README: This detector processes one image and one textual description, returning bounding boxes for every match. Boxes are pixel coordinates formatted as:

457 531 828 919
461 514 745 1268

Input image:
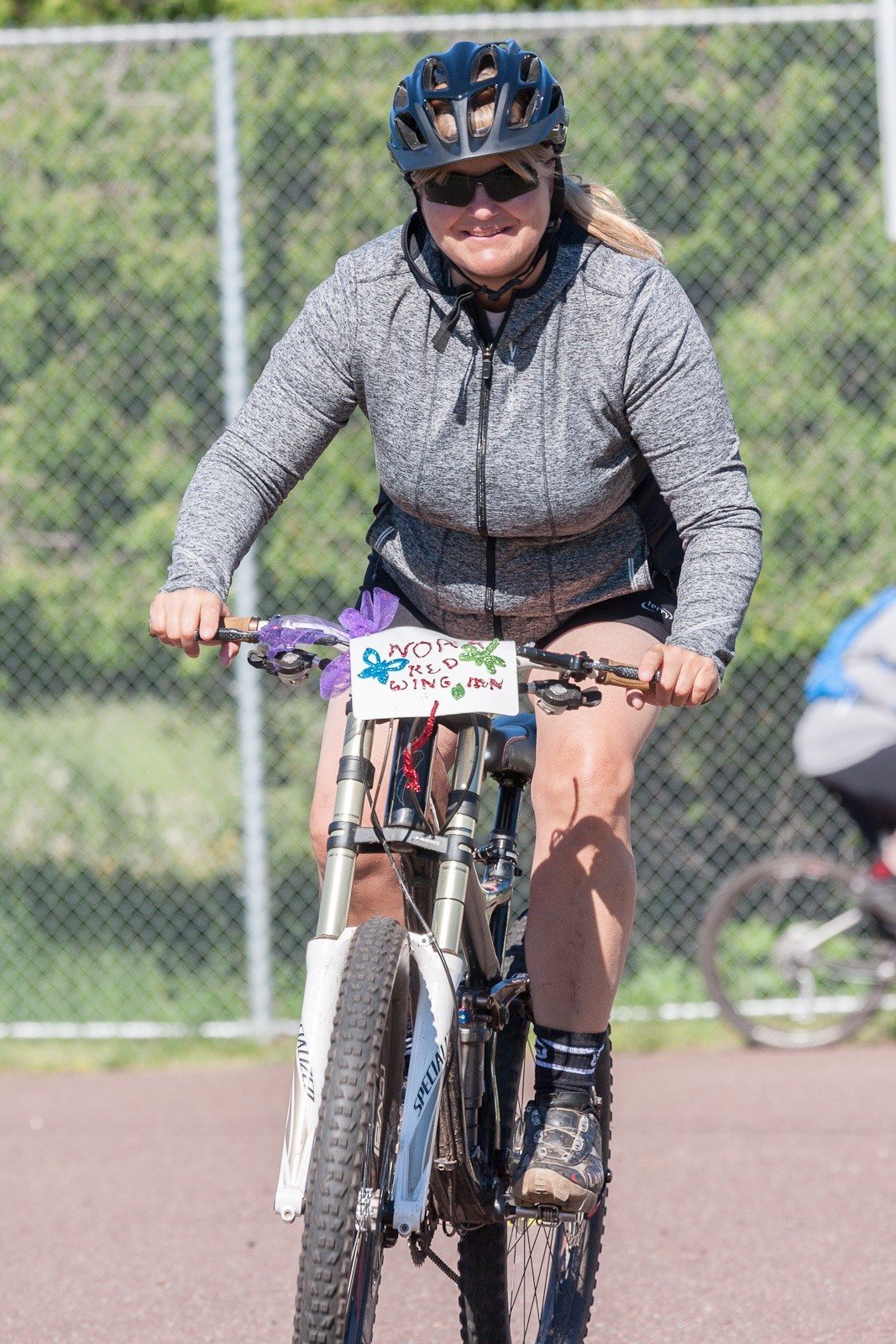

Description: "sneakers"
858 869 896 939
509 1091 606 1214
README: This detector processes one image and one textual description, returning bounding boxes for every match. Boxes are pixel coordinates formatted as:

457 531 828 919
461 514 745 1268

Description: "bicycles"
196 613 663 1344
699 852 896 1056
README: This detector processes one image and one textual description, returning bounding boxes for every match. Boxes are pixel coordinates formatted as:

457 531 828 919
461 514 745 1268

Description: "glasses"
424 156 556 207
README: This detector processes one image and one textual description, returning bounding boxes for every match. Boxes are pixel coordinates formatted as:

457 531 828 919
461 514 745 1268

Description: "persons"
149 41 763 1219
794 588 896 924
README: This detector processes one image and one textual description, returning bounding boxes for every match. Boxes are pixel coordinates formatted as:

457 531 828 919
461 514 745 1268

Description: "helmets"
384 39 570 176
